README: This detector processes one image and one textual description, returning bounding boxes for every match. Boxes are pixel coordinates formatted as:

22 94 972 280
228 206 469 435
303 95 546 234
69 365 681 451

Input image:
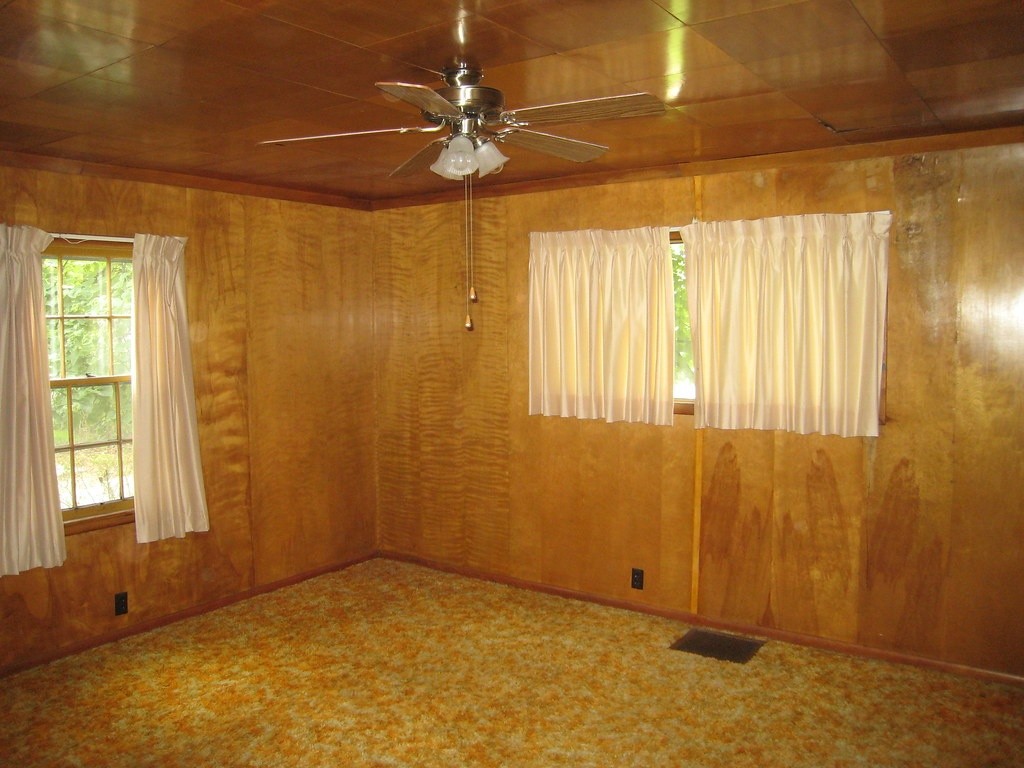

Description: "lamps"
427 119 509 184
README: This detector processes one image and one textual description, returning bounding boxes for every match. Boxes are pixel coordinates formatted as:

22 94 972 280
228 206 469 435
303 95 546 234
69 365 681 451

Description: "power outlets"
632 568 644 590
114 592 128 616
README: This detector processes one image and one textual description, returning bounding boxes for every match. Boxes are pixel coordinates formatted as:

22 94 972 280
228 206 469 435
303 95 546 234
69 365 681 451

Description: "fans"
245 69 670 182
